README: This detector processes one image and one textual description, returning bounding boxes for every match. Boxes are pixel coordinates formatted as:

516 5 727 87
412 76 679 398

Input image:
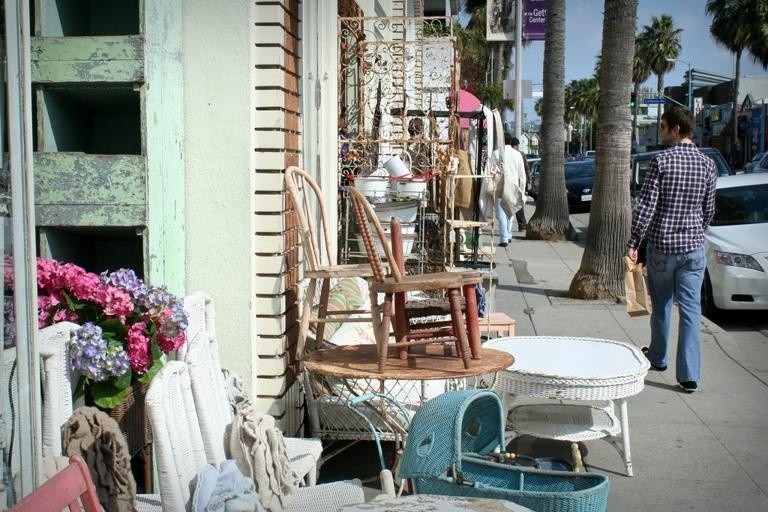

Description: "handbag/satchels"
623 256 649 317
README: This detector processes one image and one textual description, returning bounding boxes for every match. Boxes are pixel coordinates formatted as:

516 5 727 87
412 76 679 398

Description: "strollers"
349 391 612 512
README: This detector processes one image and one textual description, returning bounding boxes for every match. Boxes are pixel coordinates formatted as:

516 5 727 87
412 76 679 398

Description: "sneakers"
678 382 697 392
519 224 527 232
641 347 666 371
499 239 511 247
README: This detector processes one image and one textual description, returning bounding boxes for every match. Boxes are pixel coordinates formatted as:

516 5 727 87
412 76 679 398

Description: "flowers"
3 246 190 456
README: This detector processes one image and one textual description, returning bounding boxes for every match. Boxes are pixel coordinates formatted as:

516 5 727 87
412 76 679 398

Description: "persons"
405 118 430 168
483 133 530 247
627 104 718 391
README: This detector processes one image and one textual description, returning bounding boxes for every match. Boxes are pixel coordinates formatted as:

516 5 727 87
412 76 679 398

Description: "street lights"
569 106 593 150
666 58 692 113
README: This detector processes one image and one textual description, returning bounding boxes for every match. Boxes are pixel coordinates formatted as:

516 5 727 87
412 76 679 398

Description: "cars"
632 147 734 196
524 153 541 201
701 172 768 314
563 160 595 211
563 150 595 159
743 153 766 172
755 151 768 172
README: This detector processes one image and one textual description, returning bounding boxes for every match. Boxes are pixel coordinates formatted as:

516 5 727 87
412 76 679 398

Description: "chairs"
281 143 652 478
182 326 316 490
141 359 219 510
176 289 326 468
